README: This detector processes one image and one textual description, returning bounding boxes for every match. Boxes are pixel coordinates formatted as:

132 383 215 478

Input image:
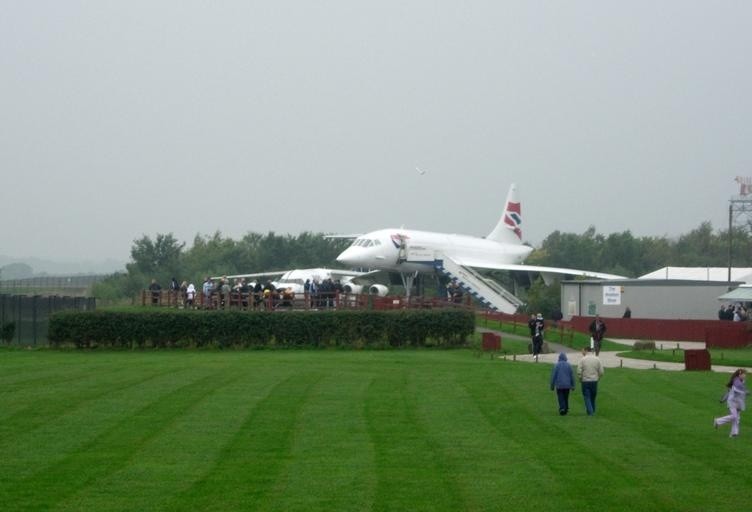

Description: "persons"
711 367 751 438
551 305 563 321
443 279 464 303
147 278 164 306
586 314 608 357
528 312 545 359
530 313 545 337
717 302 751 322
167 275 345 310
574 346 606 417
550 351 577 415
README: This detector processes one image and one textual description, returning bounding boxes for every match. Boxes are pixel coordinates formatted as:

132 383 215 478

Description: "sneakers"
729 434 739 437
713 418 719 430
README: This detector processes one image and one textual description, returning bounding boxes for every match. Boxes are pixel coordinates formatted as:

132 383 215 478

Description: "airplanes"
207 268 395 307
321 179 637 315
